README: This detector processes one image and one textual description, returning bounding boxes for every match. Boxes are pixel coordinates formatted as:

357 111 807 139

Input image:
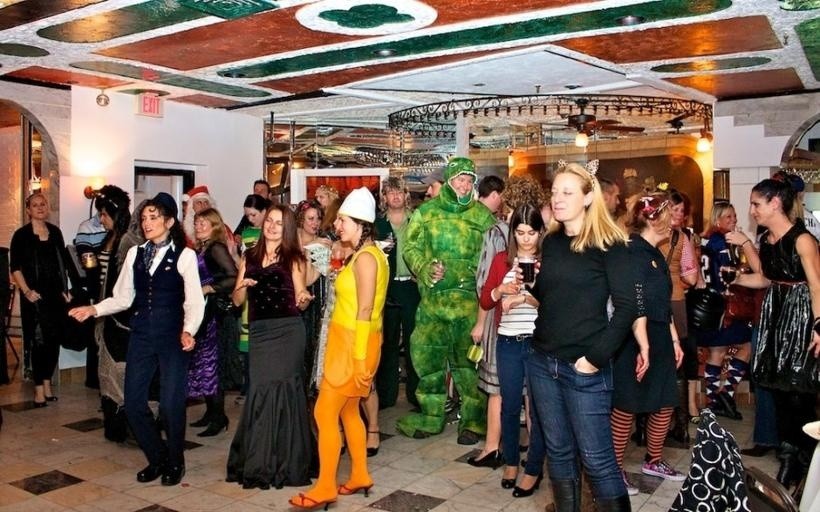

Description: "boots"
666 380 690 444
630 412 647 446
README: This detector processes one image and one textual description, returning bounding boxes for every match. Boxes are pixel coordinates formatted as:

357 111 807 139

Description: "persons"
1 150 820 510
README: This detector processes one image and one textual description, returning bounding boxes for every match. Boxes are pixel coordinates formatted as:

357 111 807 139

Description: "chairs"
741 467 799 512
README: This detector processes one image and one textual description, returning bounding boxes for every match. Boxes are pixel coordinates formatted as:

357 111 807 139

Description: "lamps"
84 175 105 199
567 122 597 147
696 117 713 152
508 150 514 168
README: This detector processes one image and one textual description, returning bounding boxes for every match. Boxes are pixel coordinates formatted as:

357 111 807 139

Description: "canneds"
83 253 93 268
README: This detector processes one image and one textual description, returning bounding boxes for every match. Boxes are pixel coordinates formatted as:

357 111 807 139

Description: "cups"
385 231 393 251
433 259 444 280
82 252 98 268
731 226 743 233
519 255 536 284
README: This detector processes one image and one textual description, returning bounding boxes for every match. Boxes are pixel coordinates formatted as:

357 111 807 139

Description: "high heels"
338 483 374 497
189 409 229 437
467 448 542 498
288 494 337 511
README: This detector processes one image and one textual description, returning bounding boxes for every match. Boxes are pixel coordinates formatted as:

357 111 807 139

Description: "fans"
530 97 644 136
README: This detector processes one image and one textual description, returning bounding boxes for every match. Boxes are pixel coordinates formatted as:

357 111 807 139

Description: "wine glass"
245 239 255 249
739 250 751 272
330 247 344 285
721 265 734 298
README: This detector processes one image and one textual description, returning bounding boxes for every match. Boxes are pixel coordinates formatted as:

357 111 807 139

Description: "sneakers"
621 470 638 496
457 430 479 444
642 459 686 481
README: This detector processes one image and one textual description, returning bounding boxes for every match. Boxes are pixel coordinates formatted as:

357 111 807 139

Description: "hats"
338 186 376 224
153 192 178 215
420 167 445 184
184 186 209 201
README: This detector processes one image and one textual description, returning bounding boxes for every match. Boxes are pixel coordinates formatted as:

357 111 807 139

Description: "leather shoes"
740 440 809 489
366 430 381 457
689 391 742 424
45 395 57 401
162 464 185 486
136 465 167 482
33 401 46 407
